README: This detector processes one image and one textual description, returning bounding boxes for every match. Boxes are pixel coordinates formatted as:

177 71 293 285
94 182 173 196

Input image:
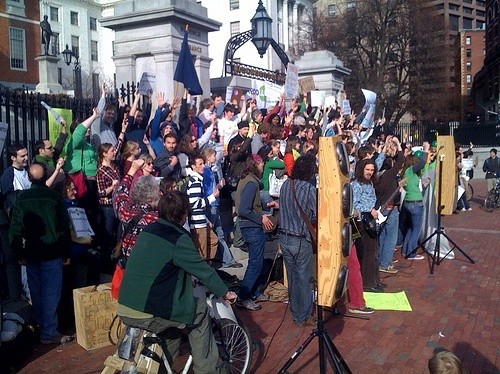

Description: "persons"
116 191 237 374
187 155 220 266
152 134 182 185
0 89 475 342
278 155 317 326
227 121 252 159
482 149 500 206
62 107 101 237
233 153 278 309
428 347 463 374
375 137 406 273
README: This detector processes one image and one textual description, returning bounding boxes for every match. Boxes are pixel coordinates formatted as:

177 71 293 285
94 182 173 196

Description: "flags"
173 30 203 95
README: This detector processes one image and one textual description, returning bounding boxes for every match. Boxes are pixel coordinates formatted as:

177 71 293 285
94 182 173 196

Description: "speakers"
436 136 458 215
318 135 350 308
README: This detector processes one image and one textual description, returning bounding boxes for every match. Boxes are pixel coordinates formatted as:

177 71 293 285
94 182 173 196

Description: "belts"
405 200 422 203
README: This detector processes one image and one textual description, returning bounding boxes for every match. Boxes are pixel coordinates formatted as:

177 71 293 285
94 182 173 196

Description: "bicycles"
484 174 500 210
118 294 254 374
465 182 475 202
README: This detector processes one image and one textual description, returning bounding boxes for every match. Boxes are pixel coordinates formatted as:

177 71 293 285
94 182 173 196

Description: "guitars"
361 177 407 238
308 221 318 255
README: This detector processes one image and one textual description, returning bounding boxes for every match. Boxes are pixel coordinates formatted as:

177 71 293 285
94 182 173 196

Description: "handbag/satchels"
66 168 87 197
268 169 288 197
263 281 289 302
224 163 238 188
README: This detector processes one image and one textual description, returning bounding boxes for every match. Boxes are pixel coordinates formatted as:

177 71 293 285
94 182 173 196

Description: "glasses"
191 138 197 143
147 162 154 166
46 146 52 150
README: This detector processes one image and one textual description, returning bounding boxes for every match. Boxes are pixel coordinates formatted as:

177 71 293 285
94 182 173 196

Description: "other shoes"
377 282 387 288
39 333 73 346
348 306 375 314
228 261 243 268
251 293 268 303
364 287 384 293
392 256 398 263
235 296 262 310
460 207 472 211
379 264 398 274
408 253 425 260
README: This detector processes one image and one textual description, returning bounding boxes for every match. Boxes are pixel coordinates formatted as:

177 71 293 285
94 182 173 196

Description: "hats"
237 121 249 129
432 140 437 146
328 110 340 122
490 148 497 155
414 149 428 162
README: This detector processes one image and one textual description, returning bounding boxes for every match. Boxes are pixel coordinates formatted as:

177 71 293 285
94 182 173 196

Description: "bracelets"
120 131 126 134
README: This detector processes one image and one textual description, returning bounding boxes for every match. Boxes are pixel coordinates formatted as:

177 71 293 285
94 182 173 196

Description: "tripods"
404 156 475 274
277 305 352 374
